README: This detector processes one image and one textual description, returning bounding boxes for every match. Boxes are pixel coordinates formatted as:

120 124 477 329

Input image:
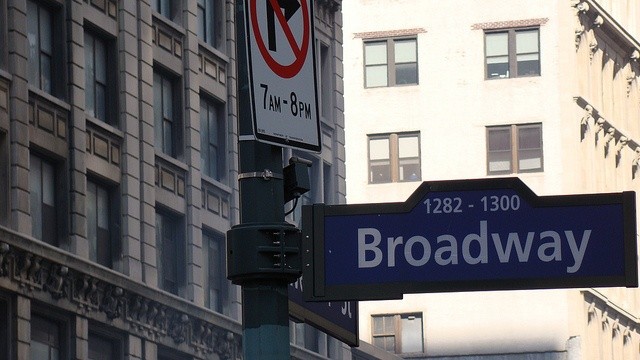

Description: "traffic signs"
286 276 359 348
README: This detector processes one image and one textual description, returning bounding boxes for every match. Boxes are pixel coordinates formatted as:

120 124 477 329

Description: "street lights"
302 177 638 302
